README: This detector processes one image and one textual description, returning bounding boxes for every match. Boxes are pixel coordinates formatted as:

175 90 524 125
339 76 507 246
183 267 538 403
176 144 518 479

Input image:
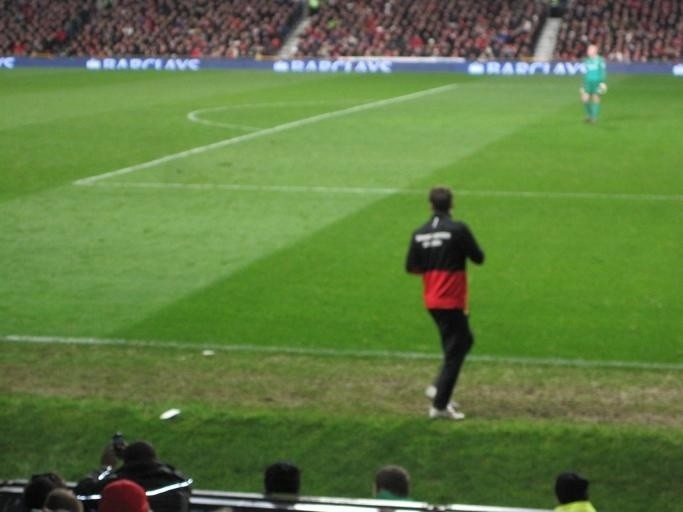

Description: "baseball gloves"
597 82 607 98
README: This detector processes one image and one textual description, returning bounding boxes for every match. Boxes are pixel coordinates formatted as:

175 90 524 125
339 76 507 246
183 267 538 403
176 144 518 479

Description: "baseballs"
203 349 214 357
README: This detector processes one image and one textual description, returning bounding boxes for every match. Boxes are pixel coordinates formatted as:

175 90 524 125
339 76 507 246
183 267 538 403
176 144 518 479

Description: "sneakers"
424 385 466 421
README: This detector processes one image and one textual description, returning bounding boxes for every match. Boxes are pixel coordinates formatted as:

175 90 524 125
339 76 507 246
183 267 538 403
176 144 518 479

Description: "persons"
404 183 485 420
552 467 598 510
262 460 301 494
0 438 193 511
576 44 607 125
0 0 683 65
370 463 412 499
